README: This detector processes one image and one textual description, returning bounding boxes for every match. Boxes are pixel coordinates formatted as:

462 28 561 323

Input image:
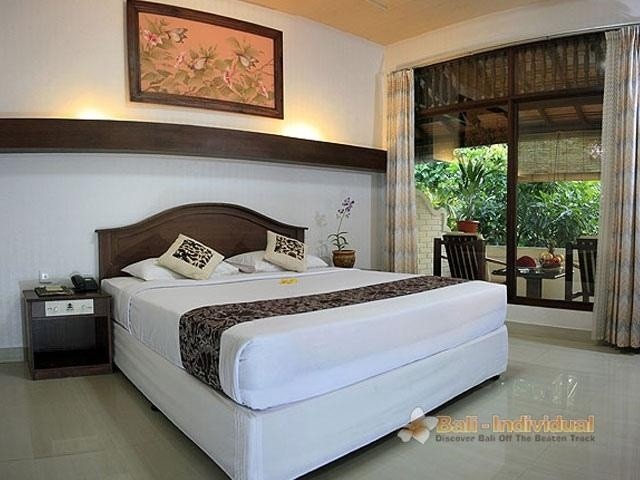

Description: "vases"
332 251 356 268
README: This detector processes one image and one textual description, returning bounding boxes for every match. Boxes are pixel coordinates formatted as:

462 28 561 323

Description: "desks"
490 264 575 299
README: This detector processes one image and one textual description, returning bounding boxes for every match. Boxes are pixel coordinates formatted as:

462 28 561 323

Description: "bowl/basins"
540 263 562 273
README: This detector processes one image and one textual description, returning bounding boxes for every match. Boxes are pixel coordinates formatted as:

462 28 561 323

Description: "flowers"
326 196 356 250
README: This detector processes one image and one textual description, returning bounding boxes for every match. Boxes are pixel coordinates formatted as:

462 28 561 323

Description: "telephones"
71 275 98 290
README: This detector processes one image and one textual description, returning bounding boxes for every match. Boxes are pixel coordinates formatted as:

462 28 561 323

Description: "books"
35 284 74 297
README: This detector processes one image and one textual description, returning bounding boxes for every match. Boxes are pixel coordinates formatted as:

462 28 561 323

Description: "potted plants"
452 156 497 232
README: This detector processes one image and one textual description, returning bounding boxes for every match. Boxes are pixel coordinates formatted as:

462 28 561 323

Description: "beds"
92 202 510 480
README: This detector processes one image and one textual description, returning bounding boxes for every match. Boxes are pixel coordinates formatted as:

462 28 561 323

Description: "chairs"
432 232 508 285
564 238 598 303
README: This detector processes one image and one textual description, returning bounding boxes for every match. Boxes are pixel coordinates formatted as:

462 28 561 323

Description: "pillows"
263 229 308 272
155 233 225 280
225 249 330 275
120 257 239 284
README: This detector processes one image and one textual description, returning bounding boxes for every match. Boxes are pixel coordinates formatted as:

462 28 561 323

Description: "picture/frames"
125 0 284 120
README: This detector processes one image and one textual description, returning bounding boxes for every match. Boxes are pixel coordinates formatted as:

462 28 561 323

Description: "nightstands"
20 287 116 380
